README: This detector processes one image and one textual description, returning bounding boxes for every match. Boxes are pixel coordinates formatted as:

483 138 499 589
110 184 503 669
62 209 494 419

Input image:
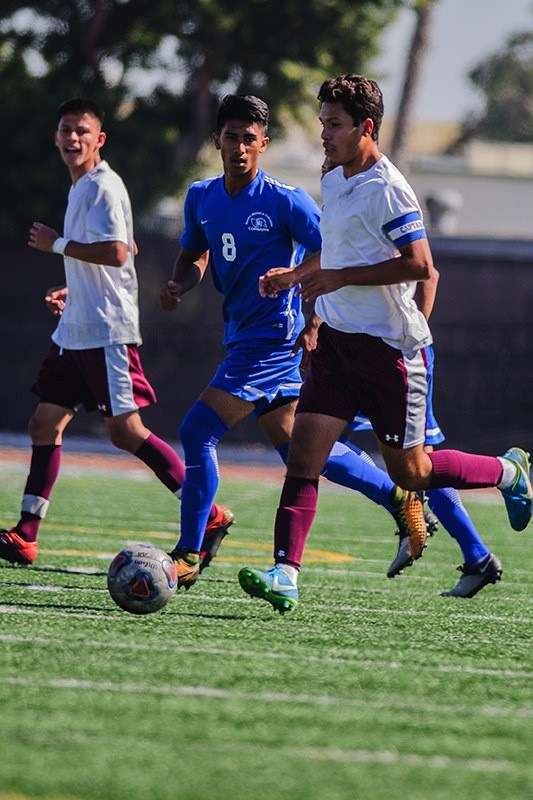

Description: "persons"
237 75 532 616
163 95 429 590
1 98 235 573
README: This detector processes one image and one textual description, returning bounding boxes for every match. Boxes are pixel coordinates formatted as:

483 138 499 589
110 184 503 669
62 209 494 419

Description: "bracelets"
51 237 70 256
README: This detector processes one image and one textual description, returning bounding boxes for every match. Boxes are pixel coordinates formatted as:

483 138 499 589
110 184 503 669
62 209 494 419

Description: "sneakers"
440 553 503 598
423 510 440 536
396 489 430 560
238 566 298 616
165 549 199 590
0 527 38 567
387 531 414 578
497 447 533 531
198 504 236 575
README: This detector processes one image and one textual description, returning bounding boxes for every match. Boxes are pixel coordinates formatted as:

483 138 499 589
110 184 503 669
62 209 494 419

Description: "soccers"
108 544 177 614
